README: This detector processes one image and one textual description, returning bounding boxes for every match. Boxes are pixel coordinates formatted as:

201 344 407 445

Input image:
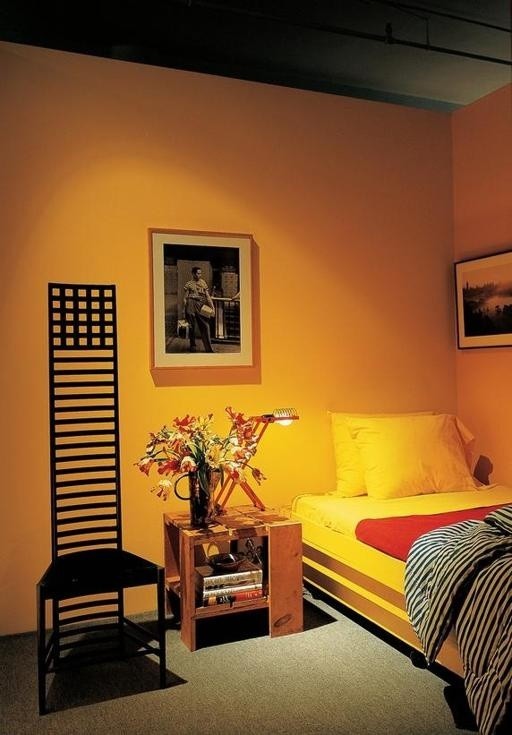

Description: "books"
195 560 263 608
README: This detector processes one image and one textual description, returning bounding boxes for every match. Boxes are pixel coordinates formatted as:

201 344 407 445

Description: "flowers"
130 406 267 524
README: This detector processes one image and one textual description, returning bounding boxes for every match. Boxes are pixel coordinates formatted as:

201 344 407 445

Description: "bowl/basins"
209 553 247 572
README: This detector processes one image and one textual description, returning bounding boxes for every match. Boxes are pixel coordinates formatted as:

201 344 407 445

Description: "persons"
183 265 217 353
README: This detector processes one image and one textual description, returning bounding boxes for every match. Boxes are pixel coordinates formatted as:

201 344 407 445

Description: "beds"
290 486 511 706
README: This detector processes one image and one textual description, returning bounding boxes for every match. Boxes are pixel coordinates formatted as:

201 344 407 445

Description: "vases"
188 466 211 529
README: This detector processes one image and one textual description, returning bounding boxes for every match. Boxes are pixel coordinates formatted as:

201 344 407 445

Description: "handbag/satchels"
176 319 190 339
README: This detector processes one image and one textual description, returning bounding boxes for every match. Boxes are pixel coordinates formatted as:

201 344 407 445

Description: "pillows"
328 409 479 501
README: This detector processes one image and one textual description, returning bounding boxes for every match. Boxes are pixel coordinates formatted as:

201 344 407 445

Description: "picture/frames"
146 226 262 388
454 247 512 350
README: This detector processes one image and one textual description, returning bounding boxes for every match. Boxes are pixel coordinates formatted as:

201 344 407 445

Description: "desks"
162 508 303 653
